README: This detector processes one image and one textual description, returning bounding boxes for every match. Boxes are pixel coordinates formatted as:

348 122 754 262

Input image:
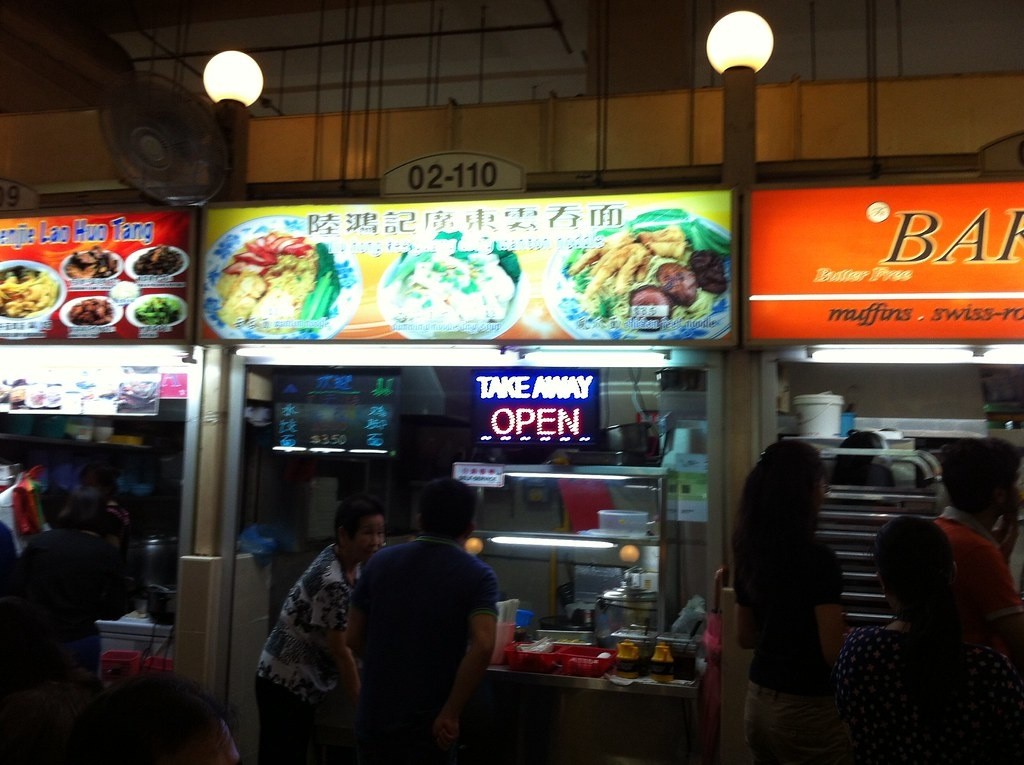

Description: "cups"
489 623 515 665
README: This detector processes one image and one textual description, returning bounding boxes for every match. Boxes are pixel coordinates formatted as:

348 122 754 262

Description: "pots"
593 579 658 649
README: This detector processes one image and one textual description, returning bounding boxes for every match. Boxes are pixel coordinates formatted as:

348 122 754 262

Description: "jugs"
616 639 640 678
650 642 674 682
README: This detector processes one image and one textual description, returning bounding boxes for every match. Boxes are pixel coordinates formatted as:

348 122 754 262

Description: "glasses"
813 483 831 499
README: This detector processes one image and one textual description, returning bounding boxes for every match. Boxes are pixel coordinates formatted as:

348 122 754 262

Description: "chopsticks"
495 598 520 623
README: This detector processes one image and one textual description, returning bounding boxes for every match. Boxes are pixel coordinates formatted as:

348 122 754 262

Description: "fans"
97 68 229 208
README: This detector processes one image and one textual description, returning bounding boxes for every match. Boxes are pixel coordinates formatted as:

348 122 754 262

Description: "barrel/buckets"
793 394 844 436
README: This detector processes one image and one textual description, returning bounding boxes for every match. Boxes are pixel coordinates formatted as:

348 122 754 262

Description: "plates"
202 215 363 339
374 252 532 340
123 245 189 281
58 296 124 327
59 250 123 281
543 218 732 340
125 293 189 327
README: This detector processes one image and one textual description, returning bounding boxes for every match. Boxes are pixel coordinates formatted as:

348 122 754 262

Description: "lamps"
200 49 266 203
706 11 774 190
488 535 619 550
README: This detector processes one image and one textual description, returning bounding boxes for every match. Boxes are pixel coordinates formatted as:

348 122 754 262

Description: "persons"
729 436 1024 764
254 476 502 765
61 676 245 765
0 497 131 677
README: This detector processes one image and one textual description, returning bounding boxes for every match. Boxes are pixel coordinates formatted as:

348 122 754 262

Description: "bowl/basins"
840 412 857 437
606 422 647 453
93 426 115 443
598 510 649 537
0 260 67 340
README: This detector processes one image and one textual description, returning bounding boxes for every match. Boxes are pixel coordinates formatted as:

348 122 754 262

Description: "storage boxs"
974 364 1024 403
536 629 594 643
143 656 176 680
610 627 660 675
505 641 569 673
102 674 123 688
468 465 671 632
982 403 1024 414
556 646 618 678
656 632 702 682
660 453 710 524
597 509 649 533
988 420 1024 429
990 429 1024 447
984 412 1024 422
99 650 140 675
